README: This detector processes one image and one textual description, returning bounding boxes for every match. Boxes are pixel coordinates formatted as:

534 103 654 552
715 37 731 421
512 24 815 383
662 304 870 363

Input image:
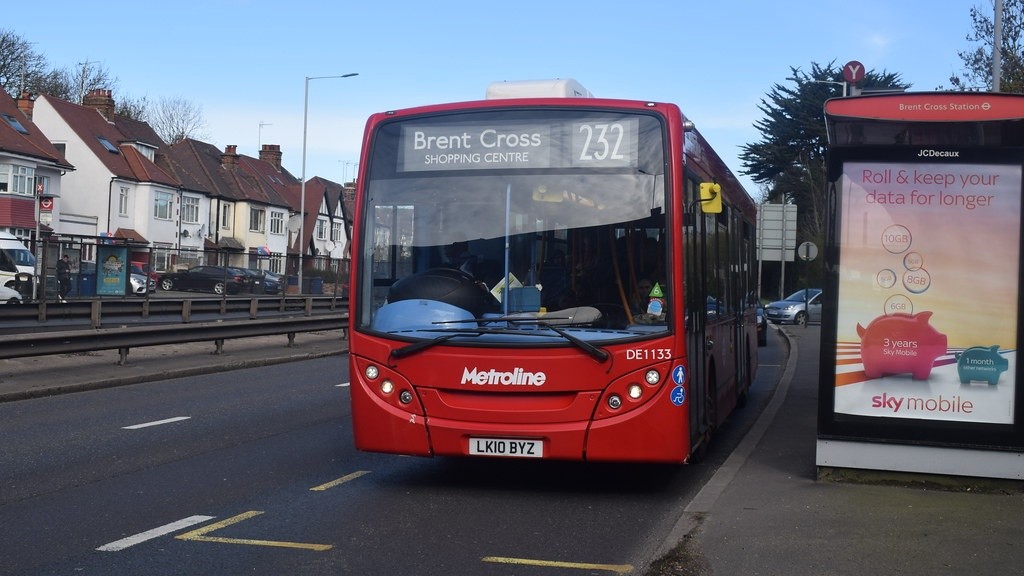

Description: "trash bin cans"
302 278 323 294
15 273 33 300
65 272 95 297
241 276 264 294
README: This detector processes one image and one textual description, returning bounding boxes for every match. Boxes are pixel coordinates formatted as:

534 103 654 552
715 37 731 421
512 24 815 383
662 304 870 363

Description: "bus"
348 78 758 471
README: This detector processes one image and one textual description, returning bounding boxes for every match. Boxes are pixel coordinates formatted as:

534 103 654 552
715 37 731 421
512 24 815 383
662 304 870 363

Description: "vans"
0 232 38 290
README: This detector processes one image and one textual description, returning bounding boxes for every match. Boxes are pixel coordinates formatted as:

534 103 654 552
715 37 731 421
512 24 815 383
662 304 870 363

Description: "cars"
764 289 822 326
224 267 283 295
81 261 157 296
0 286 23 304
128 262 157 282
158 265 254 295
757 299 768 346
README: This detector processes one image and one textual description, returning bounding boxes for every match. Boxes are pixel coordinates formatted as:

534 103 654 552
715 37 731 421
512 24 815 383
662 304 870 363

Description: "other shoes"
58 295 62 302
60 299 67 303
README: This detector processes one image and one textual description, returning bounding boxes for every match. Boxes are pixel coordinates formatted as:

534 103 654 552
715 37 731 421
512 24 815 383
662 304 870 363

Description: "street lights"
298 72 359 295
786 77 846 97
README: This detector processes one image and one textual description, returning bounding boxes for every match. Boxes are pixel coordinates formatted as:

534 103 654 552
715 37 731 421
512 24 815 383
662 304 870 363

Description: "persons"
550 248 566 265
56 255 72 303
444 233 485 279
638 272 653 302
